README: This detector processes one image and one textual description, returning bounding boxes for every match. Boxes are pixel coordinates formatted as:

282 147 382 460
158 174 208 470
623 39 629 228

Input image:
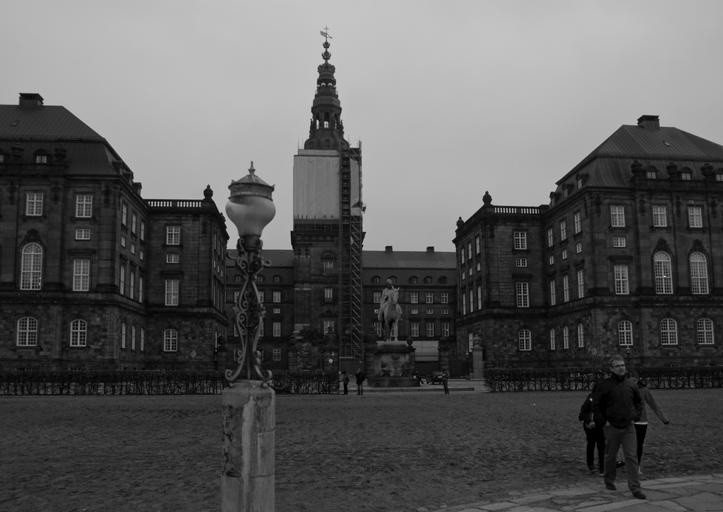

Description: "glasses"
614 364 624 366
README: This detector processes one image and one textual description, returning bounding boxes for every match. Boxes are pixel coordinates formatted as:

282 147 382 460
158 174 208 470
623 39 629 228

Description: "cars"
425 370 448 385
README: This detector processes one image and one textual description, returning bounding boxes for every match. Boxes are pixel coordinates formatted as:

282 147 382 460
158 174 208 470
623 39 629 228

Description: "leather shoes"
606 481 616 490
633 491 645 499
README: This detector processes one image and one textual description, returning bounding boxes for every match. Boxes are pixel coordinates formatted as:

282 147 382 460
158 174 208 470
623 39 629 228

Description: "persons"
339 367 365 395
378 279 404 322
579 353 669 499
442 369 450 395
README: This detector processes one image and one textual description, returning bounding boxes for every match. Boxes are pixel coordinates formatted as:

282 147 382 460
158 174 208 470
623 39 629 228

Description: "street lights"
216 159 276 511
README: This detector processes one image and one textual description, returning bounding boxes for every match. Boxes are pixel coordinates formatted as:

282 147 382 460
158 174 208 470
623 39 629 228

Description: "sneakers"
591 461 643 477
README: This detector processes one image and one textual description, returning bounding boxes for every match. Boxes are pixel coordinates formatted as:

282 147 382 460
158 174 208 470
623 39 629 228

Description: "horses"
383 285 403 342
